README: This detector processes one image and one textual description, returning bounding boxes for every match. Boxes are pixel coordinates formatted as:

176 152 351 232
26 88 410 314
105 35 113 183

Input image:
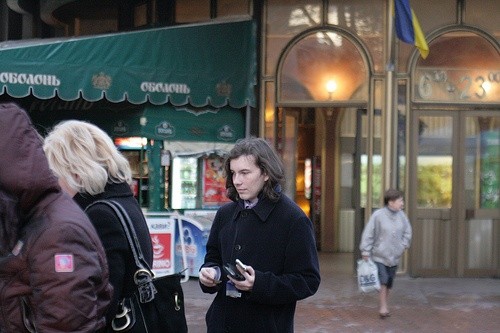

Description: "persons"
43 120 153 333
359 189 413 317
0 104 115 333
199 137 321 333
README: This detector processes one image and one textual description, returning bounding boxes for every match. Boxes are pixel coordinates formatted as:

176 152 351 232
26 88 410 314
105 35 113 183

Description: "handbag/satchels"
357 258 381 294
83 198 189 333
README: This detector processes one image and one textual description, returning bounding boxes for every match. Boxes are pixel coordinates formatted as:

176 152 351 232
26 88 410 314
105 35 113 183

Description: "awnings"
0 17 258 109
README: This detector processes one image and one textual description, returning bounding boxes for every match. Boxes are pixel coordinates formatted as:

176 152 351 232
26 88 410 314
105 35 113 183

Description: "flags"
395 0 429 60
